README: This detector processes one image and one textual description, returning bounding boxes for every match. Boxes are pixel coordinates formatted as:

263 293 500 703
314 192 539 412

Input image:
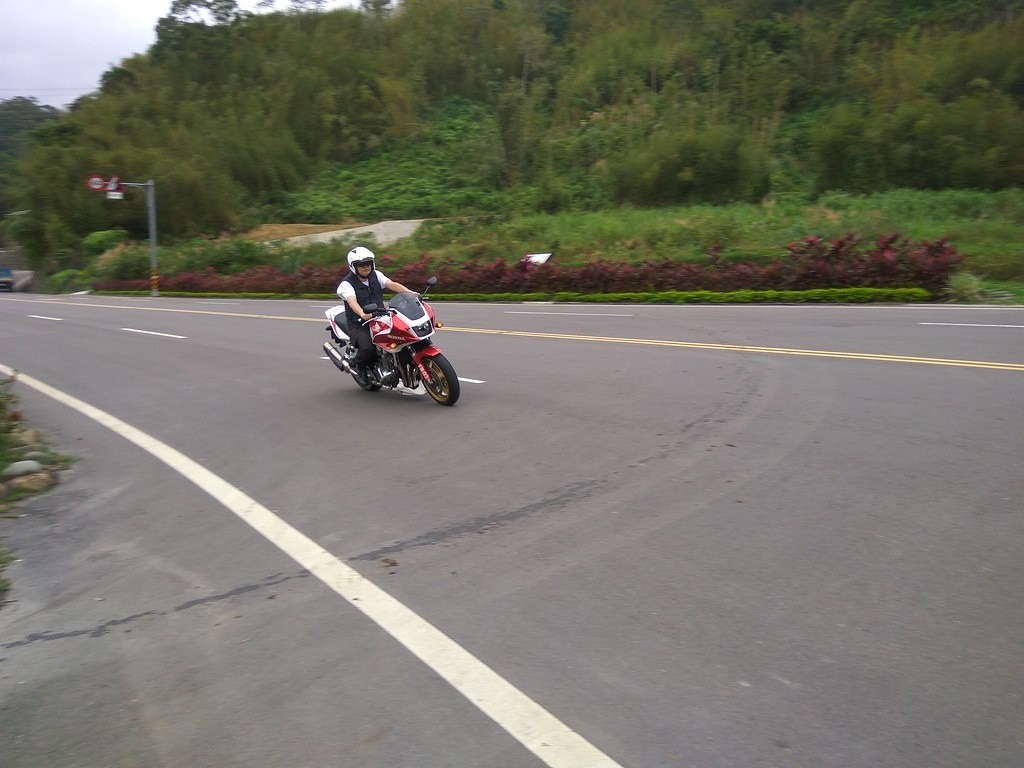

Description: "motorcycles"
324 276 460 407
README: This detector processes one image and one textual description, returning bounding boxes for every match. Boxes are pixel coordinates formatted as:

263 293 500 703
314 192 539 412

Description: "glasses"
355 262 373 267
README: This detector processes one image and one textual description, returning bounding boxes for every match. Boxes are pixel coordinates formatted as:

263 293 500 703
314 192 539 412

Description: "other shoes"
356 364 369 386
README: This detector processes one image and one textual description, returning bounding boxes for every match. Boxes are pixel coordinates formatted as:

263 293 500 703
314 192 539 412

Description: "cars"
0 268 13 291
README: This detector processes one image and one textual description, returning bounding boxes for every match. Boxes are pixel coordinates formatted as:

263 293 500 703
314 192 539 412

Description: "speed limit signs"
89 176 104 190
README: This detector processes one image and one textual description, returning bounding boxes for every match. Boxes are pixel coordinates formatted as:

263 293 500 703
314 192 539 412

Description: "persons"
336 246 421 384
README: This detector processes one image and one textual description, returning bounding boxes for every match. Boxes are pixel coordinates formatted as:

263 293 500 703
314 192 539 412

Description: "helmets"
348 246 376 274
